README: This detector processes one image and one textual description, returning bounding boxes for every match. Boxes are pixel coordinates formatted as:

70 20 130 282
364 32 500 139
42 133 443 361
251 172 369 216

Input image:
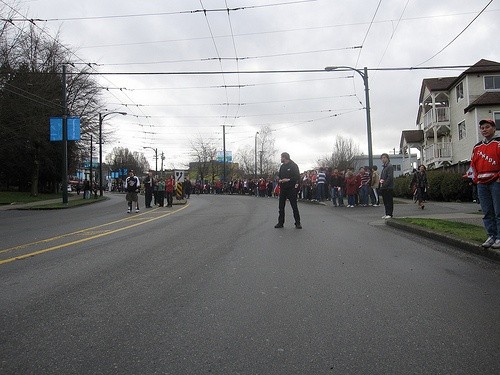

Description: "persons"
408 164 427 210
60 165 379 208
378 153 394 218
126 169 141 214
274 152 303 229
468 118 500 250
462 166 481 205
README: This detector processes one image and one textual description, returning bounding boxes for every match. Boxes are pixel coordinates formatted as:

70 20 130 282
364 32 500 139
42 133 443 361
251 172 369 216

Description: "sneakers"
491 240 500 248
482 235 494 247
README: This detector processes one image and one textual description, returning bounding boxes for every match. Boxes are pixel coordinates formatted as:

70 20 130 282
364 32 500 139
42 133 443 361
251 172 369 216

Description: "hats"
479 119 496 127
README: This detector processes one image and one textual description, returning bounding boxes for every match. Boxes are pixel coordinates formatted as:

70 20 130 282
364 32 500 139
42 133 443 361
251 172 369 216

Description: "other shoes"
135 210 139 212
364 204 367 206
350 204 356 207
373 204 380 207
346 205 350 207
385 215 391 219
358 204 361 207
127 210 132 212
361 204 364 207
274 223 283 228
382 215 386 218
296 224 302 229
368 204 372 206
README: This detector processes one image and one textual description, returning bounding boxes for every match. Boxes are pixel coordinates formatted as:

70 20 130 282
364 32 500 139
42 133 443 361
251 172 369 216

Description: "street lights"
325 65 373 171
98 111 127 197
255 131 260 173
144 146 158 174
